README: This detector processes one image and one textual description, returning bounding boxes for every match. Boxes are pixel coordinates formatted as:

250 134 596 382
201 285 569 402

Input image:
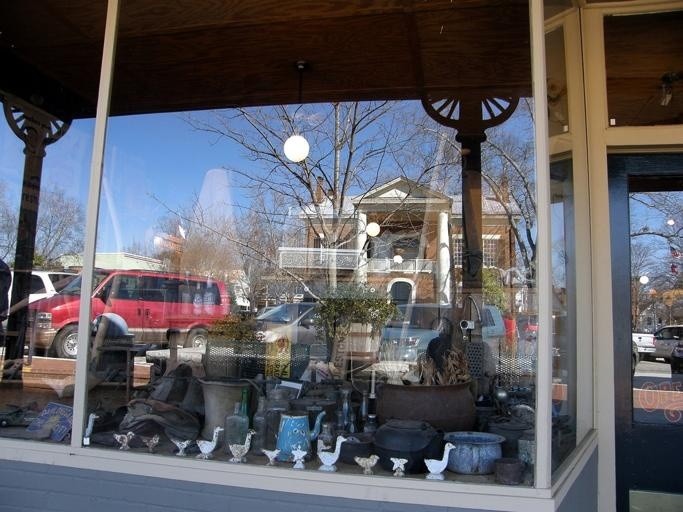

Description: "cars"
236 302 324 346
631 323 682 375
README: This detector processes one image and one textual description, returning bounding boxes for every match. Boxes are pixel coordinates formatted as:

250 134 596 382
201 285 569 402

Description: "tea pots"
265 408 327 464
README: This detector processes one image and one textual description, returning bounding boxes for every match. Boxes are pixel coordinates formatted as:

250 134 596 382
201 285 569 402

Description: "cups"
491 458 529 485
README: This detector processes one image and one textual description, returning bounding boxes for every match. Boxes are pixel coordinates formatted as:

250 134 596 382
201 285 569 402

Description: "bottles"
316 385 379 465
225 389 289 456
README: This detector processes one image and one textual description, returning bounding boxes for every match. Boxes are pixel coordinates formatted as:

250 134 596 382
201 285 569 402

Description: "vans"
2 267 80 331
377 302 538 365
22 265 231 359
